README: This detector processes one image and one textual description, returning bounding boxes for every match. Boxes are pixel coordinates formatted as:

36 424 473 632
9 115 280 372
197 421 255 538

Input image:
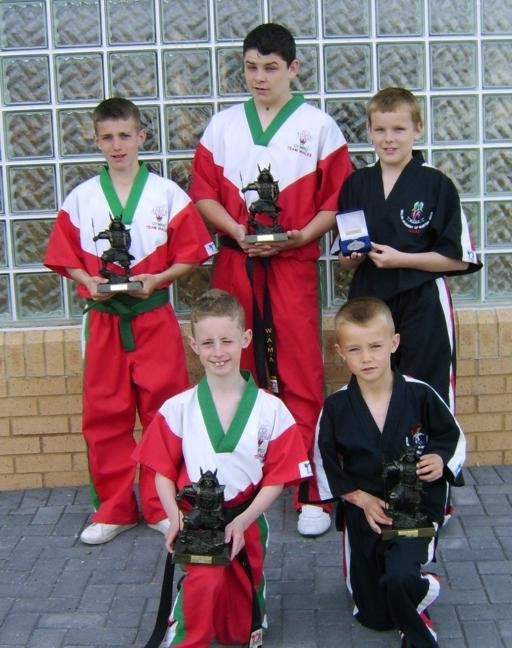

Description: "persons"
89 210 147 292
184 24 358 540
313 293 469 648
378 446 437 542
236 160 291 244
130 285 318 647
43 96 219 547
332 86 485 413
168 468 233 566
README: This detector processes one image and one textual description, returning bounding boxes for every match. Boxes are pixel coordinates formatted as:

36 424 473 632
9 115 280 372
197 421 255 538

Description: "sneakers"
297 504 332 536
147 519 171 536
80 522 138 544
